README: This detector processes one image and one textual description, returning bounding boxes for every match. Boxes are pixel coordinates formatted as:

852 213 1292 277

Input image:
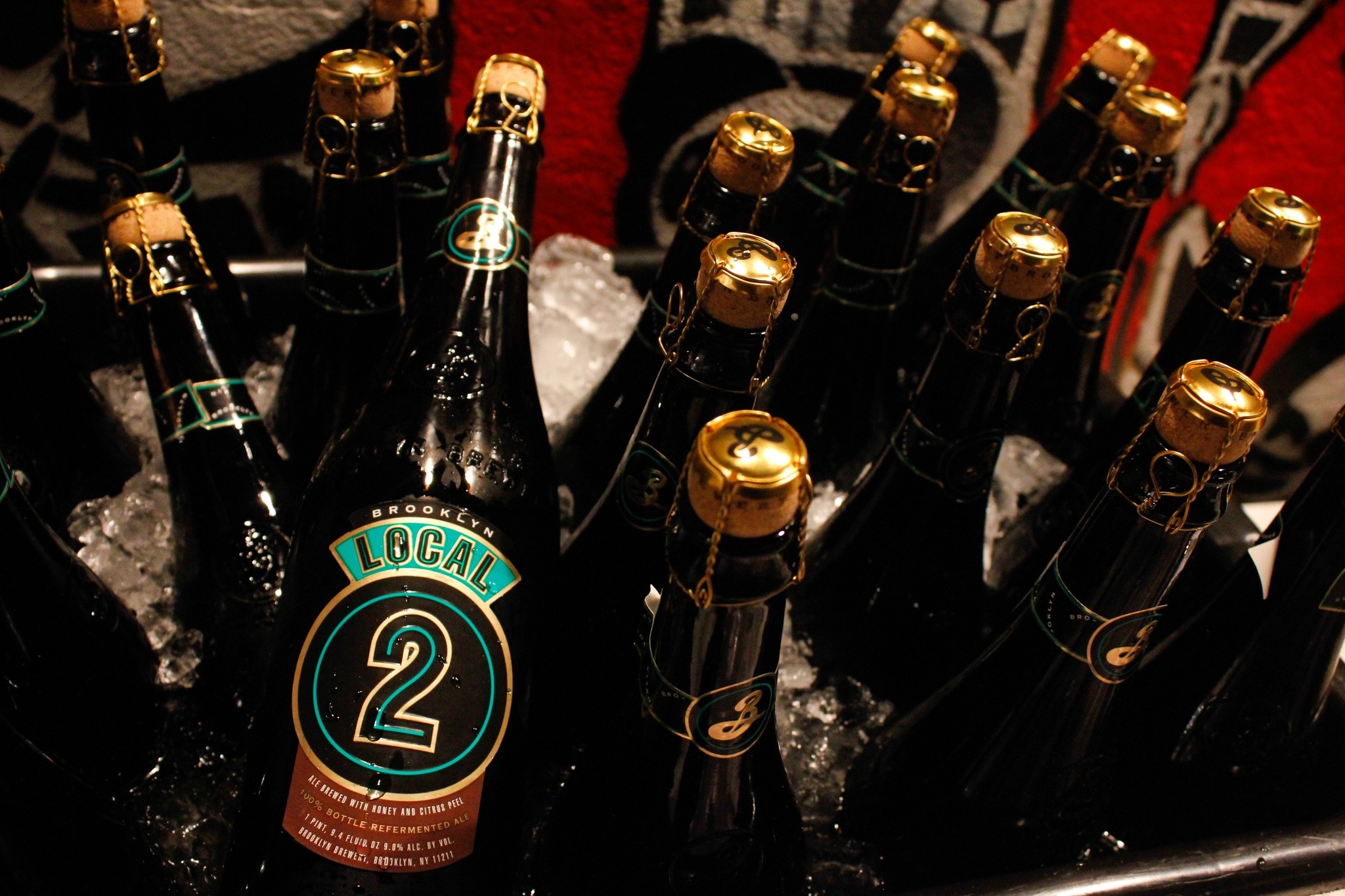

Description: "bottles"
769 72 959 495
369 0 458 264
527 410 804 896
1 214 144 552
1 460 159 792
259 52 556 896
770 14 962 292
984 184 1323 821
1009 84 1190 473
104 190 297 728
837 355 1269 896
1075 404 1344 869
874 28 1161 469
803 211 1070 734
558 229 795 768
64 3 222 264
552 109 795 538
